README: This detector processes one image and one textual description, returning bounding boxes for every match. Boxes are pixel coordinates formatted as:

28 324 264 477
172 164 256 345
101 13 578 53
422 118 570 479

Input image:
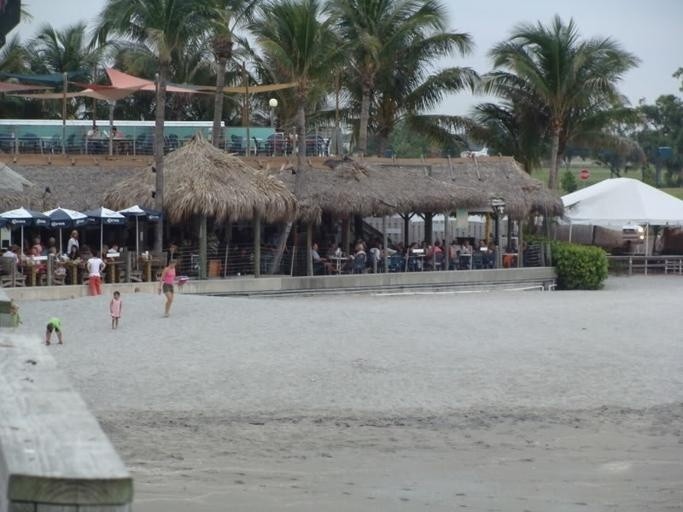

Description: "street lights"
267 98 279 127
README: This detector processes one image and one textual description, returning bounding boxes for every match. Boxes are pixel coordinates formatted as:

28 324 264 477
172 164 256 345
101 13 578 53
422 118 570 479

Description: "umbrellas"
116 205 164 269
0 204 48 275
41 205 89 256
83 205 128 259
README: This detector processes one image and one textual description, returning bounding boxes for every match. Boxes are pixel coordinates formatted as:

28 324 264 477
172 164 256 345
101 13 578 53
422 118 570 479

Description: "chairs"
0 252 518 288
0 132 296 158
665 259 682 275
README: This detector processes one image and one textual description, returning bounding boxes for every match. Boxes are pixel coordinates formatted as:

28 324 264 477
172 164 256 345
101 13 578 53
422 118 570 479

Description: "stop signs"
579 169 589 182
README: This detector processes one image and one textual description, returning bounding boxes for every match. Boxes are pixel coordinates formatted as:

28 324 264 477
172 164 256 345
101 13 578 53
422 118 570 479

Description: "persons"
109 291 123 329
106 126 126 153
86 250 106 297
8 300 24 328
87 126 103 153
157 260 188 317
44 317 63 345
